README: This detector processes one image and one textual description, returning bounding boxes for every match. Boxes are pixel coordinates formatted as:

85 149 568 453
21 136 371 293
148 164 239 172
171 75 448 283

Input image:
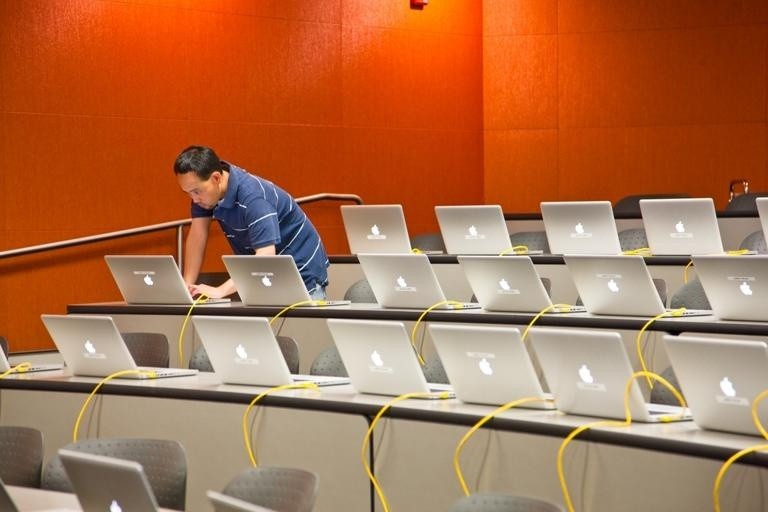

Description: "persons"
173 145 331 301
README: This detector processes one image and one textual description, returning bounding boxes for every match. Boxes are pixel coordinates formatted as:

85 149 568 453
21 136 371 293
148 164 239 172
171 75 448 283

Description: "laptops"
222 254 351 307
540 200 623 255
57 448 161 512
434 204 543 255
691 254 768 322
326 318 456 400
528 327 693 423
561 254 712 317
203 486 273 512
0 343 62 373
191 315 351 387
40 314 199 379
103 255 231 305
662 335 768 437
356 252 481 309
340 204 443 254
456 255 586 312
639 198 758 256
428 324 557 410
